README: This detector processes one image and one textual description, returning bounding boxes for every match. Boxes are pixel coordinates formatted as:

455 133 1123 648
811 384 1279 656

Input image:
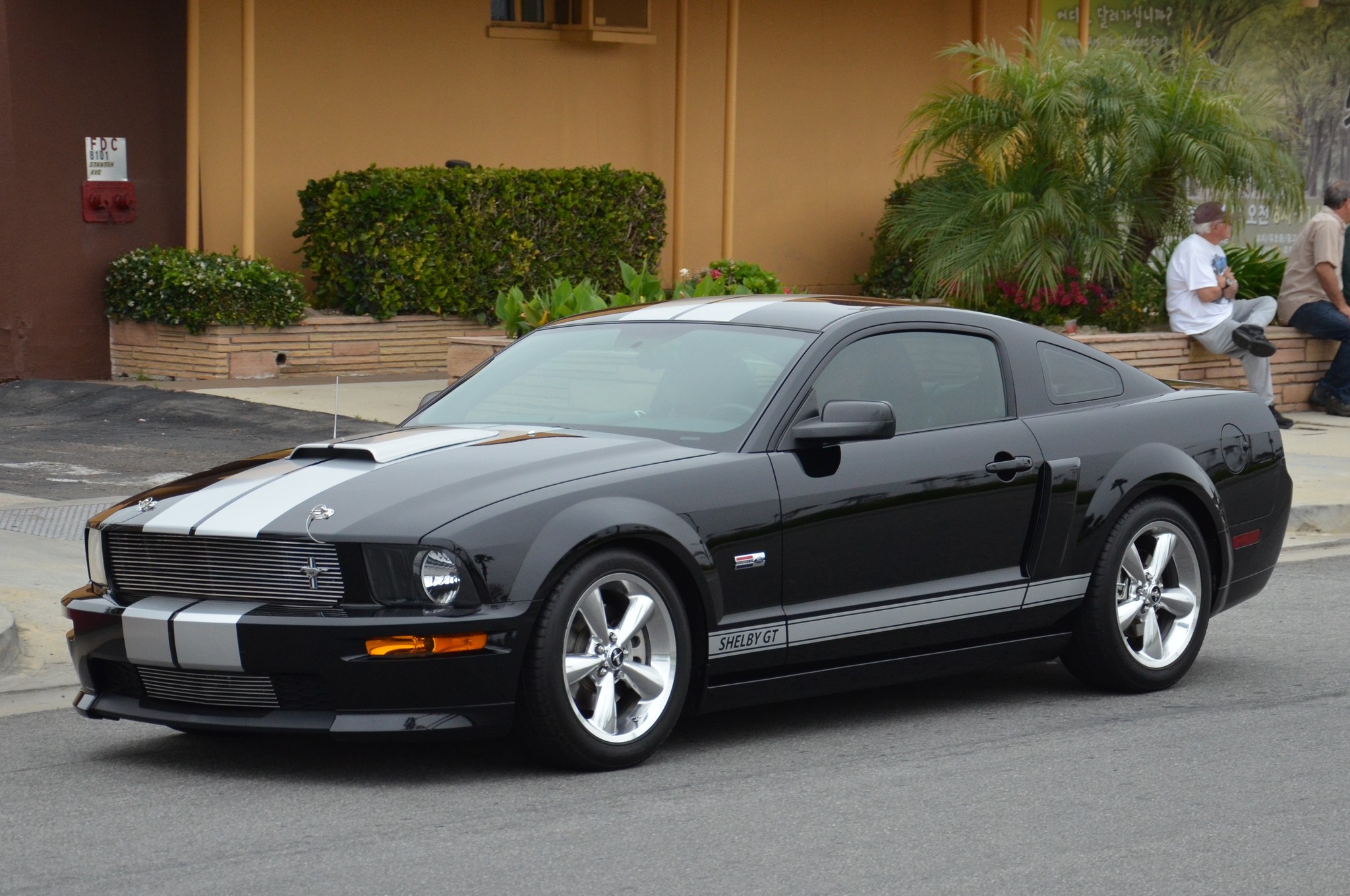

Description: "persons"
1276 180 1350 417
1166 202 1295 428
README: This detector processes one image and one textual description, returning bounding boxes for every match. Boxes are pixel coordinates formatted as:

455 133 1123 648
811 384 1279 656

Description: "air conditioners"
552 0 651 33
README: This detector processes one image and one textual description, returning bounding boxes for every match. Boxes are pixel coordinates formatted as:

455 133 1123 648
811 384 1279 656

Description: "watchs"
1222 277 1232 290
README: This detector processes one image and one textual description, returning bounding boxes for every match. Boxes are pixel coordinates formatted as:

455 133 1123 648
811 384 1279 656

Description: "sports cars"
61 292 1295 772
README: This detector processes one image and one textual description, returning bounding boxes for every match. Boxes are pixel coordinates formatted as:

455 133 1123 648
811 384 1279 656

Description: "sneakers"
1268 402 1294 429
1232 324 1277 357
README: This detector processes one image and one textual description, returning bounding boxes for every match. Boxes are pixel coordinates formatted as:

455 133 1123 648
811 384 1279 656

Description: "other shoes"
1308 383 1350 417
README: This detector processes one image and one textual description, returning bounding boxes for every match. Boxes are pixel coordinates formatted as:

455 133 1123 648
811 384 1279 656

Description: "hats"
1193 201 1242 224
